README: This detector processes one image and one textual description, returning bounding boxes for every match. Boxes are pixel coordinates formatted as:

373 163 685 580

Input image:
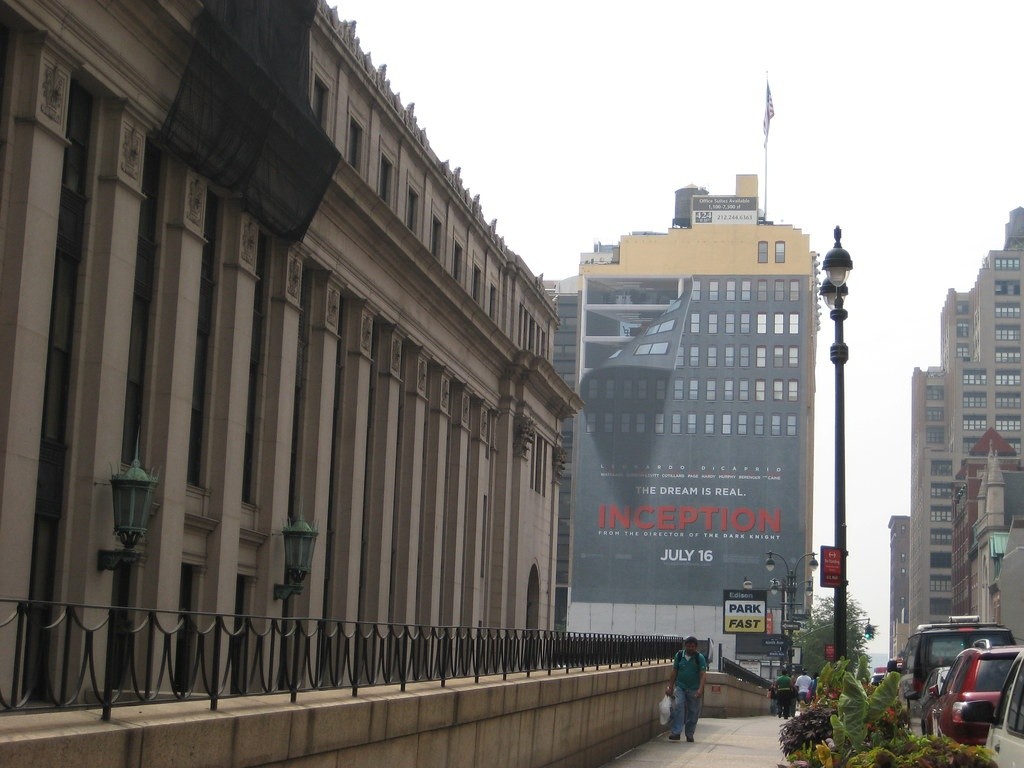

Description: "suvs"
929 639 1024 748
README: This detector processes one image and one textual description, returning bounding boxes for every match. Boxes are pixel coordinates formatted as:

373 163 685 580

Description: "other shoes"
687 735 694 742
669 733 680 740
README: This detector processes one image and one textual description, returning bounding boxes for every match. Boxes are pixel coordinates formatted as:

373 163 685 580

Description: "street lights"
766 549 818 676
820 225 854 676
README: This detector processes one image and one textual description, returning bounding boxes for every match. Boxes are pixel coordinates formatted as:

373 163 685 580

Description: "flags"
763 81 776 145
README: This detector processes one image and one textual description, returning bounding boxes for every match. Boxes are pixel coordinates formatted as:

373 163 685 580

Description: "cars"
904 666 951 738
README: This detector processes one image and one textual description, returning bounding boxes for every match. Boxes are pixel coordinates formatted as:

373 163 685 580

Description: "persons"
767 665 819 720
667 636 707 742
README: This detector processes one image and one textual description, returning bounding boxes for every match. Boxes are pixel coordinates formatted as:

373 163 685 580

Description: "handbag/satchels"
659 694 672 725
767 690 771 697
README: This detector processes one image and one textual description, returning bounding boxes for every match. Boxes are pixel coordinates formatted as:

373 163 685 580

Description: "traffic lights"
865 625 871 640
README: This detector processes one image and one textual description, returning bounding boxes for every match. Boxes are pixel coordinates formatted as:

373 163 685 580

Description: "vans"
887 616 1017 738
